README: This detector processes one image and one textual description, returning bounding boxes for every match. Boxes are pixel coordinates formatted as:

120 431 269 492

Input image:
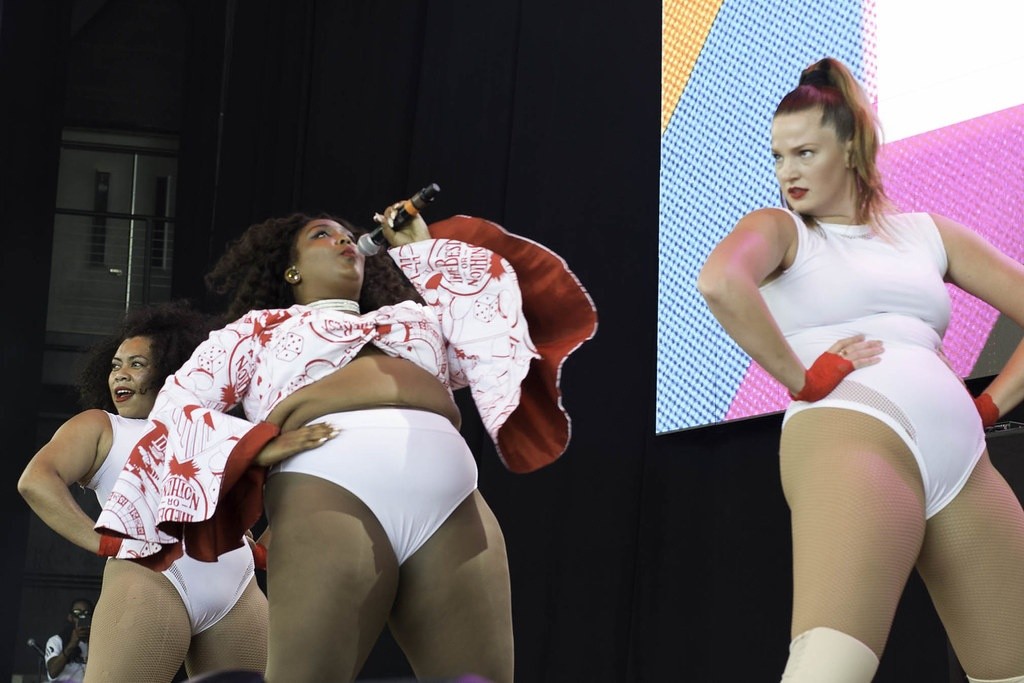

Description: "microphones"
358 184 440 257
27 639 45 658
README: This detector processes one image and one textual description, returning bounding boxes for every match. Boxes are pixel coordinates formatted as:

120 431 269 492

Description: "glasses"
70 608 93 616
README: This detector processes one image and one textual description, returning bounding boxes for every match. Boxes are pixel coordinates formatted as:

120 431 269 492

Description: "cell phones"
78 615 91 628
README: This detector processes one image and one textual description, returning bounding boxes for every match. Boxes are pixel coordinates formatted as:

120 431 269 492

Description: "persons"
94 197 599 683
695 57 1024 683
17 301 268 683
